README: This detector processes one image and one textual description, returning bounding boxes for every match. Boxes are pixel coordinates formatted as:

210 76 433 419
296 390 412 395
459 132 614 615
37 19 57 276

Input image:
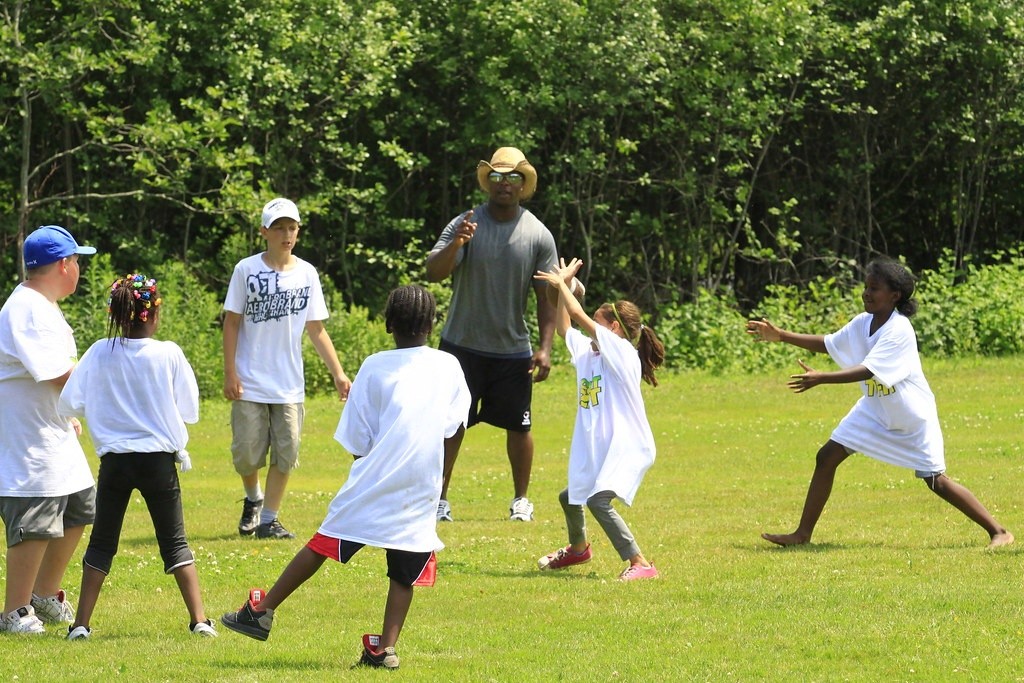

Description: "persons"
0 226 97 635
530 257 659 580
57 273 219 640
426 146 559 522
746 262 1015 550
220 198 352 540
221 285 472 672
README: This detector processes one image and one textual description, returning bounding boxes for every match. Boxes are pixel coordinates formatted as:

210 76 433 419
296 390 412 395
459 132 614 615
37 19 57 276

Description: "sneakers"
0 606 45 633
618 562 659 581
238 497 263 536
220 589 273 641
256 519 295 539
537 543 591 568
436 499 451 520
509 497 533 521
31 589 76 625
351 635 398 670
64 624 92 640
189 618 217 639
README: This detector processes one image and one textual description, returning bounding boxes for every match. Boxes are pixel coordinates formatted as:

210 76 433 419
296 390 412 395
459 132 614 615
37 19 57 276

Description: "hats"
261 198 300 229
477 147 537 200
23 224 97 266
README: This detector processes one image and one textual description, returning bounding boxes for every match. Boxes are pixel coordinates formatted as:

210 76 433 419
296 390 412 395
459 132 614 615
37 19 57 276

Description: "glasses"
486 171 524 184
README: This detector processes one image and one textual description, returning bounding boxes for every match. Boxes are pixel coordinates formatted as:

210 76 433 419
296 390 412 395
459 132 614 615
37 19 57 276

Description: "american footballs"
546 273 588 312
